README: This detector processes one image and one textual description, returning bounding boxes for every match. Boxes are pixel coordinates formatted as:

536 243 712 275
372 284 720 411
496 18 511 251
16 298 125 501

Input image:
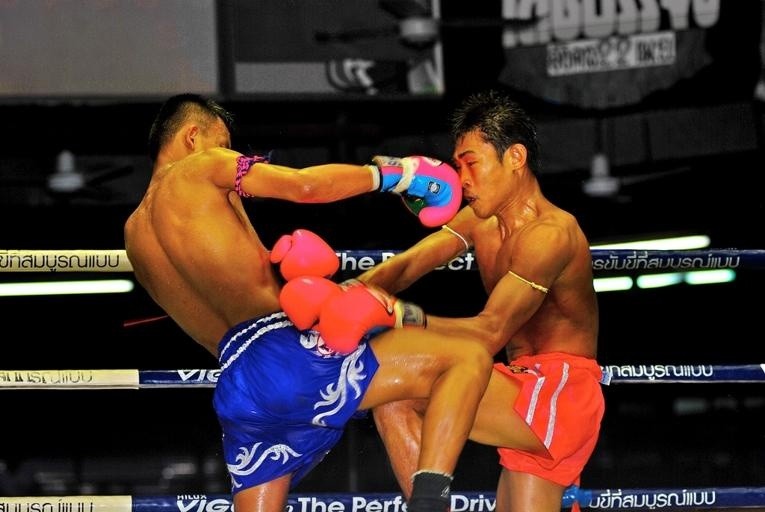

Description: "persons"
278 90 607 512
123 93 463 512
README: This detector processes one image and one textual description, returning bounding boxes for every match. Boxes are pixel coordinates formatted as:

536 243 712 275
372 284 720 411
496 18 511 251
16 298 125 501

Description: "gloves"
279 275 367 332
271 229 340 282
366 154 463 227
320 286 427 355
